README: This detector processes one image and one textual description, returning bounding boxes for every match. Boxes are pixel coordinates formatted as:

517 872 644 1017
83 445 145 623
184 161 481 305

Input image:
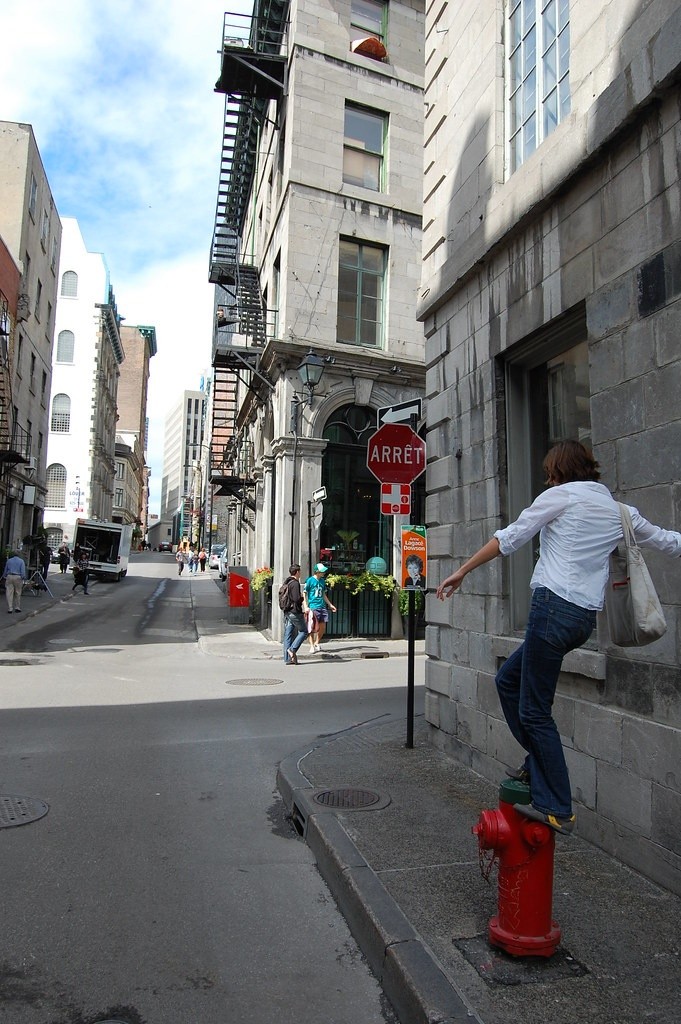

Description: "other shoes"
83 592 90 595
7 611 13 614
287 648 297 665
14 609 21 613
286 661 296 666
310 646 315 654
513 803 576 836
72 585 75 590
314 644 321 652
504 766 531 786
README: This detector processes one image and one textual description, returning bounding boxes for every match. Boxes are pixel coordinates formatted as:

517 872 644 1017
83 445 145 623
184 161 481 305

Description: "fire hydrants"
472 779 561 959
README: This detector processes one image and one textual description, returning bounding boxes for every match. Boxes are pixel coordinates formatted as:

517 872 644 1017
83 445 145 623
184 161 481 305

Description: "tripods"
22 551 55 599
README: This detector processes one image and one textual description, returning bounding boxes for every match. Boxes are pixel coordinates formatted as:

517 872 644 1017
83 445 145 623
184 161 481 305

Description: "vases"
332 583 355 591
266 577 272 586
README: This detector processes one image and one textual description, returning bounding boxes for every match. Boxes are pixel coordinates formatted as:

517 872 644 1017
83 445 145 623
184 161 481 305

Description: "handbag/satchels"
303 609 320 634
604 501 666 648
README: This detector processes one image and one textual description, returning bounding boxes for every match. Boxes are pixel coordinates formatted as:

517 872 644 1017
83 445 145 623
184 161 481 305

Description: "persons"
279 563 309 664
40 542 52 584
303 562 336 653
186 548 194 573
0 550 26 613
404 554 425 589
72 551 90 595
189 550 199 576
198 548 207 572
436 440 681 836
57 542 71 573
175 548 188 575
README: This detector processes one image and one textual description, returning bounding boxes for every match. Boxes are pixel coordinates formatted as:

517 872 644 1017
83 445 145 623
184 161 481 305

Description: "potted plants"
398 588 422 640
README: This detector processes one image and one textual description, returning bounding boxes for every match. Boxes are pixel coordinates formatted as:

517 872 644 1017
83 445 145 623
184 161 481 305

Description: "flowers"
323 573 356 591
250 567 273 591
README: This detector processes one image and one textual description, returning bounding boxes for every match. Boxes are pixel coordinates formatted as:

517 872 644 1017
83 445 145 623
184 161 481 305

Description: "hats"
314 563 328 573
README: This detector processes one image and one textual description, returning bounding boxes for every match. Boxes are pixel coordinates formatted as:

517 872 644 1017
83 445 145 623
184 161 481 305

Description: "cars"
158 542 172 552
50 542 73 564
206 545 228 582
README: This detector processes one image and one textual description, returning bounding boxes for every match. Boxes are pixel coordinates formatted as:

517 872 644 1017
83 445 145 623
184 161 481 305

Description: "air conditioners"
24 457 37 470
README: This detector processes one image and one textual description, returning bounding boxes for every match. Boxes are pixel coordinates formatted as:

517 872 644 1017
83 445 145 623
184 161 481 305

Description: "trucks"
69 518 133 582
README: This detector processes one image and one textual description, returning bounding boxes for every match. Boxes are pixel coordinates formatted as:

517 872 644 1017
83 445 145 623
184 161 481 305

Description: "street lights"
183 465 202 552
188 442 211 558
181 496 193 541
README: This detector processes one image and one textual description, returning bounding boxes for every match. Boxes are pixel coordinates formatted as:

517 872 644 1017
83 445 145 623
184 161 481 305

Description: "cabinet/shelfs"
321 549 366 572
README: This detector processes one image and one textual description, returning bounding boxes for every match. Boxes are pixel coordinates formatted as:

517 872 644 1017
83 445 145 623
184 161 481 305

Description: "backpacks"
279 580 300 611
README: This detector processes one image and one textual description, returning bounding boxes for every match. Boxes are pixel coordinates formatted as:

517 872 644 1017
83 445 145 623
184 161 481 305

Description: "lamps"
290 347 326 431
